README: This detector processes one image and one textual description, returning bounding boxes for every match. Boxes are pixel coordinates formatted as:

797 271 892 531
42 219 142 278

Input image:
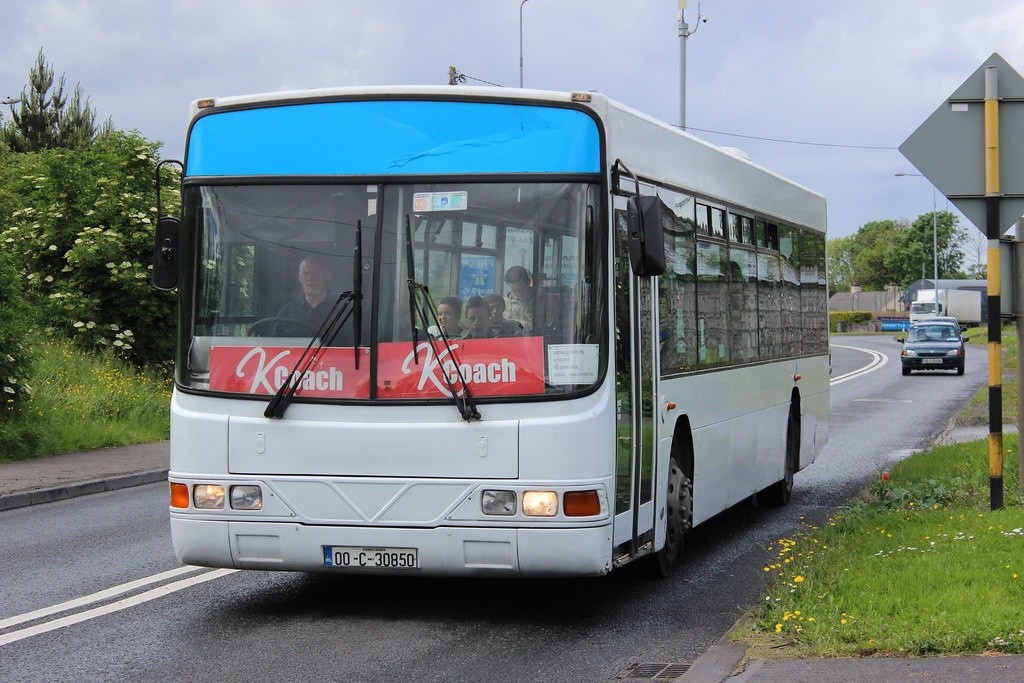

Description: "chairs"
487 277 570 337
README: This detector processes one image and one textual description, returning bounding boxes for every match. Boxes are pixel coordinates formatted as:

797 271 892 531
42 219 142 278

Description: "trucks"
908 288 983 332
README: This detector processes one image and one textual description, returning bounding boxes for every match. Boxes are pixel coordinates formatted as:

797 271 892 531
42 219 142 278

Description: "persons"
461 296 501 339
481 292 522 338
427 296 471 340
266 252 363 346
500 264 562 344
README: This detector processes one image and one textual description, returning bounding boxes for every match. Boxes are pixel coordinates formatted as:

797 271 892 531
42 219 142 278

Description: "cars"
896 316 969 376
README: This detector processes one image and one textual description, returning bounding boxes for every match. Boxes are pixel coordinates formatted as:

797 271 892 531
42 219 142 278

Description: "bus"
150 79 830 592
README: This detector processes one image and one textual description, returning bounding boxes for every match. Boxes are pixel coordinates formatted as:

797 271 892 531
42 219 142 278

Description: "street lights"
893 172 940 315
